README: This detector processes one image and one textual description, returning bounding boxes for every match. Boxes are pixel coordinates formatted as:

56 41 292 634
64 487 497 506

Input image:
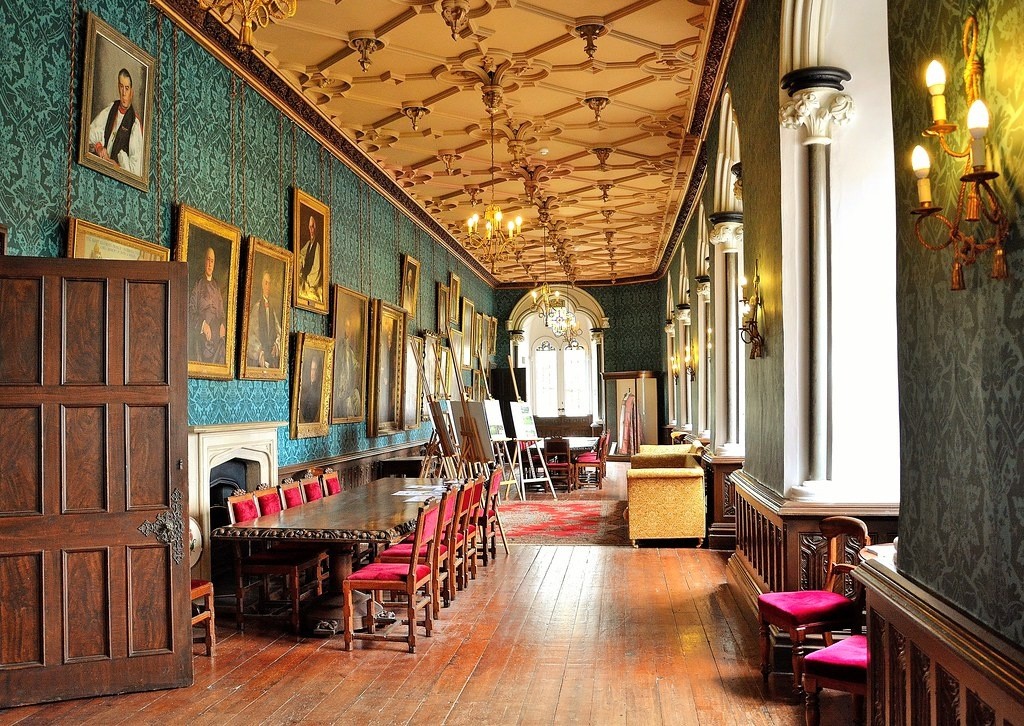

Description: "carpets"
490 498 629 545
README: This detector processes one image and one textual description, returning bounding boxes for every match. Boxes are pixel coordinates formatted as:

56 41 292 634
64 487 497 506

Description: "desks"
380 457 438 476
521 437 599 489
860 540 895 564
210 477 491 636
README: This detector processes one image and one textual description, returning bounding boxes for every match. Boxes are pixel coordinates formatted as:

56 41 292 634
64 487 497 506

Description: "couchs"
626 441 709 549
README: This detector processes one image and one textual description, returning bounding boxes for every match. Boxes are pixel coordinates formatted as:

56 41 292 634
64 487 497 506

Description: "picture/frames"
67 9 499 440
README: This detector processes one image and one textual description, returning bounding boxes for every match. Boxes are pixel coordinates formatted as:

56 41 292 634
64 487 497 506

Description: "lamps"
552 272 584 339
466 98 526 269
686 344 695 381
670 356 680 385
738 260 765 361
910 15 1008 291
527 205 562 327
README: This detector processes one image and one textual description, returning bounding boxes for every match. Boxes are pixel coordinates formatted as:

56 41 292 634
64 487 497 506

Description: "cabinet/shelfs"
601 371 661 462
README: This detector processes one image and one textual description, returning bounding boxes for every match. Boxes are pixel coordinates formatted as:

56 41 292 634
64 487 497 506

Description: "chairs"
758 514 869 726
514 429 611 493
189 469 503 652
